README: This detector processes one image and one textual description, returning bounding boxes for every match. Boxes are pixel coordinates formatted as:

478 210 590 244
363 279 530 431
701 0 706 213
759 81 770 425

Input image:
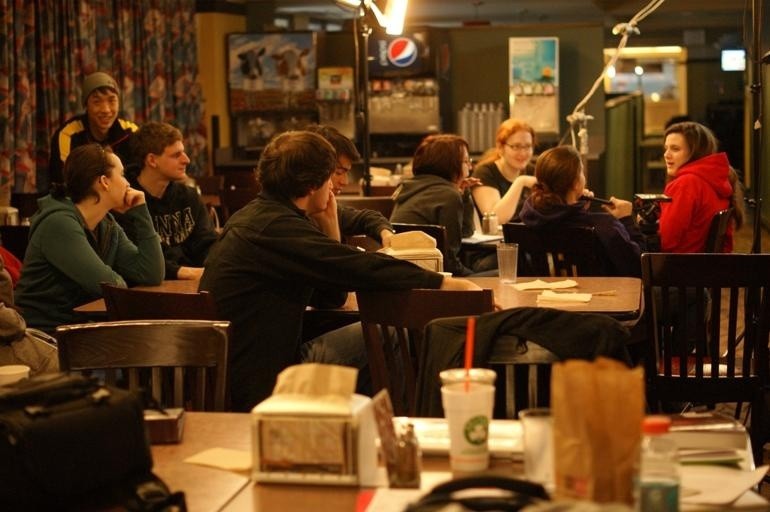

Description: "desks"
149 410 760 512
73 276 644 322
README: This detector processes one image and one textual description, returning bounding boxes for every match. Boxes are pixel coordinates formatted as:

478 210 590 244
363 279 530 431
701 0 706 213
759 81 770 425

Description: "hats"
81 71 118 106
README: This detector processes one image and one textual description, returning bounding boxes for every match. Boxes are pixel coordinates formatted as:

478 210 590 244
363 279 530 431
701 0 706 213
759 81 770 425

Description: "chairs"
0 175 770 466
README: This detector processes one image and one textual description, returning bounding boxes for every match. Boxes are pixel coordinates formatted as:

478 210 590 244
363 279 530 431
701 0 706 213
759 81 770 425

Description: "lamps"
333 0 408 196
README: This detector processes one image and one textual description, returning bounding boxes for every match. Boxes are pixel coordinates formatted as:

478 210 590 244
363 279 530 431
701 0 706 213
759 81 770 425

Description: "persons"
470 116 537 233
519 144 712 360
49 72 139 197
656 122 747 253
110 121 221 281
388 132 497 276
1 256 58 375
14 143 166 377
197 129 504 412
299 122 394 248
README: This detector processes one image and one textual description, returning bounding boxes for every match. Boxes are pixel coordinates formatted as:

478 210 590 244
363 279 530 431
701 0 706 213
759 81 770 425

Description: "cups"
454 99 505 154
495 242 519 284
516 409 558 487
437 362 499 473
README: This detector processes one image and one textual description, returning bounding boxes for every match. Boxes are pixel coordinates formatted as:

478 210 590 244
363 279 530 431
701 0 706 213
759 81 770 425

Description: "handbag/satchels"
0 376 187 512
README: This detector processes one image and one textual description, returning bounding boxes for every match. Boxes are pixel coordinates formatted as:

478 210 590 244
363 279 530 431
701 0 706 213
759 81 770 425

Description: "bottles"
630 415 684 511
21 217 31 226
387 422 422 487
0 206 18 227
481 212 500 237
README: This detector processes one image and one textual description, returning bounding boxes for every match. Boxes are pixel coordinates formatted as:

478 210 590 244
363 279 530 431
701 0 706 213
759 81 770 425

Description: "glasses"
505 142 532 150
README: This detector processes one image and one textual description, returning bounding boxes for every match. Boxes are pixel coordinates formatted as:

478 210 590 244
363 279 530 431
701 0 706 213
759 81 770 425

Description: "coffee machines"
366 25 449 157
223 31 322 159
